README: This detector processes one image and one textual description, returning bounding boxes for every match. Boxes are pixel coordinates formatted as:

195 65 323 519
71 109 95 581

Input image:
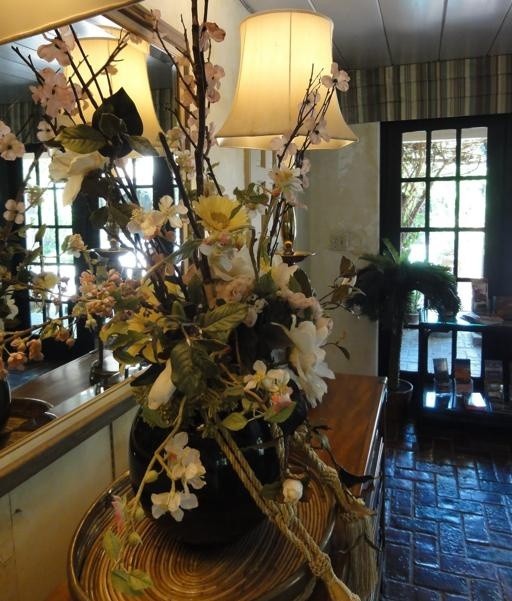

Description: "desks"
64 450 343 601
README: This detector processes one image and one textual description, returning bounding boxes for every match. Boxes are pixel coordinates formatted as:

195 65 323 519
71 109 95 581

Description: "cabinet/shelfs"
416 302 512 441
8 351 117 411
303 368 392 601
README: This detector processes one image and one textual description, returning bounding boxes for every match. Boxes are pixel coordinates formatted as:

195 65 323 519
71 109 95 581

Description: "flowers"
65 59 366 551
1 23 141 382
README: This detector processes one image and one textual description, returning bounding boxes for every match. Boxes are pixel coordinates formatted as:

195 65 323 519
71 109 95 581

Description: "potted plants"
335 239 458 437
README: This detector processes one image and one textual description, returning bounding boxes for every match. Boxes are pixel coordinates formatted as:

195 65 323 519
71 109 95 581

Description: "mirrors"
1 0 192 499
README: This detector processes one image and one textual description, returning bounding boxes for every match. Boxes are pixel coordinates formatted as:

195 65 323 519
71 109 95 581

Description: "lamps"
214 8 361 249
61 36 173 287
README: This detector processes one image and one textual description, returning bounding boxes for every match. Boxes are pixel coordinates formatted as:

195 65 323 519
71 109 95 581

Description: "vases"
127 327 289 552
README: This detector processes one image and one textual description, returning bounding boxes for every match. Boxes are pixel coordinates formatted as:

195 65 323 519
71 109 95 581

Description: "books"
461 312 505 327
